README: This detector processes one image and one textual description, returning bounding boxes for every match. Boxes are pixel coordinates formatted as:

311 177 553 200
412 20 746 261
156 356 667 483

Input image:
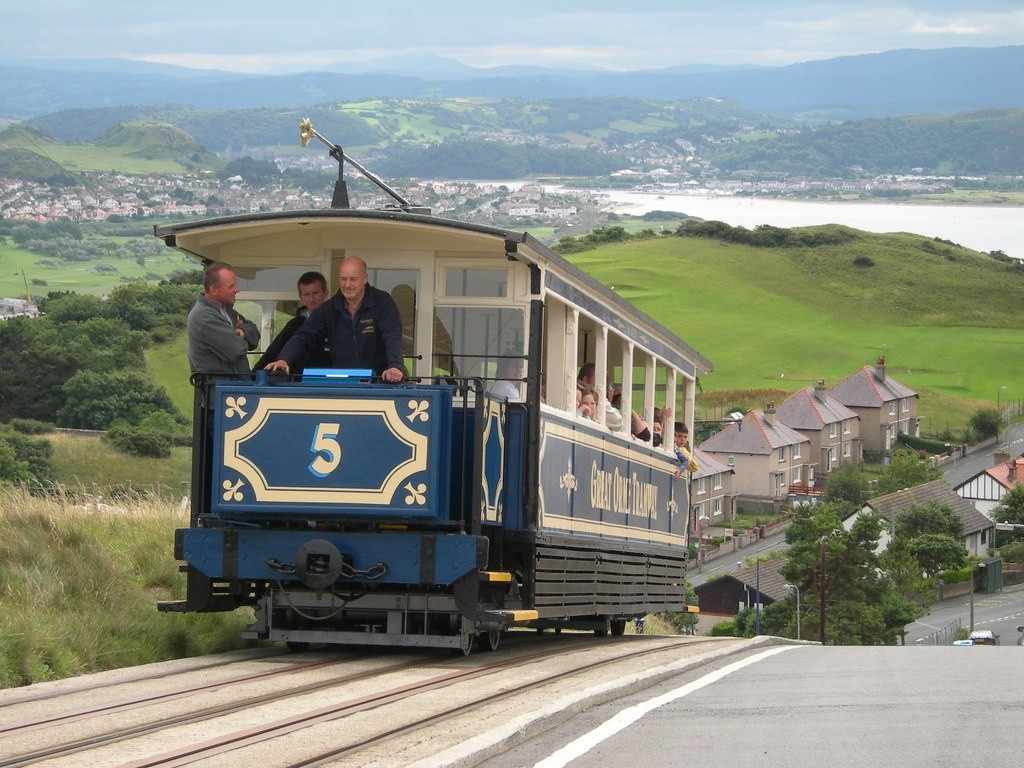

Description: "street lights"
782 584 801 640
995 385 1007 444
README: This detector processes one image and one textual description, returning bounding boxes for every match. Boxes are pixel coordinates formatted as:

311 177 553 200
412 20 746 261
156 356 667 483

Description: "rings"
394 378 397 380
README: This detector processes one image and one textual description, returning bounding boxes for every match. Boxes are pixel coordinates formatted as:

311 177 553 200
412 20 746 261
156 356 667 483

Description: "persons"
252 271 332 383
540 378 547 404
186 262 261 440
489 350 523 399
390 283 459 376
576 362 697 480
263 255 404 383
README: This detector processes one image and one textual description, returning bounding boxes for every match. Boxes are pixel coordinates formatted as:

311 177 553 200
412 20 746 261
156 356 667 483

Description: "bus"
154 116 715 656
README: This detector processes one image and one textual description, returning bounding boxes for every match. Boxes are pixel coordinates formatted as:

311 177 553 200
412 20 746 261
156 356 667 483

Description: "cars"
968 629 1000 645
952 639 974 647
